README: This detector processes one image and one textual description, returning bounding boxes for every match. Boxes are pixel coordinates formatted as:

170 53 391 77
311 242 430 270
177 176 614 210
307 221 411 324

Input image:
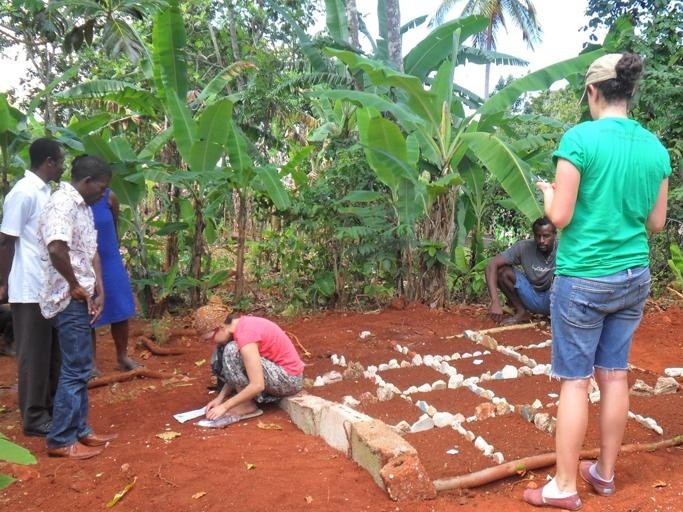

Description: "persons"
193 294 302 420
523 54 673 511
485 217 558 327
0 139 65 436
71 154 146 375
0 292 16 358
37 155 118 459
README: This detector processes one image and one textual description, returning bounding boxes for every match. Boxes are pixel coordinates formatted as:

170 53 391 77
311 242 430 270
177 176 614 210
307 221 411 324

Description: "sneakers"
523 485 583 511
574 53 638 108
578 460 616 497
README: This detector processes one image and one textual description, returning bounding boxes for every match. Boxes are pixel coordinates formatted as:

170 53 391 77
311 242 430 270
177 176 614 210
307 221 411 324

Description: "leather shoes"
46 439 103 461
22 420 55 437
79 432 112 446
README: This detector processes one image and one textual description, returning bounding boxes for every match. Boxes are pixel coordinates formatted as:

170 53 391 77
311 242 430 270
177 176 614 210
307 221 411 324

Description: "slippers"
113 362 144 373
